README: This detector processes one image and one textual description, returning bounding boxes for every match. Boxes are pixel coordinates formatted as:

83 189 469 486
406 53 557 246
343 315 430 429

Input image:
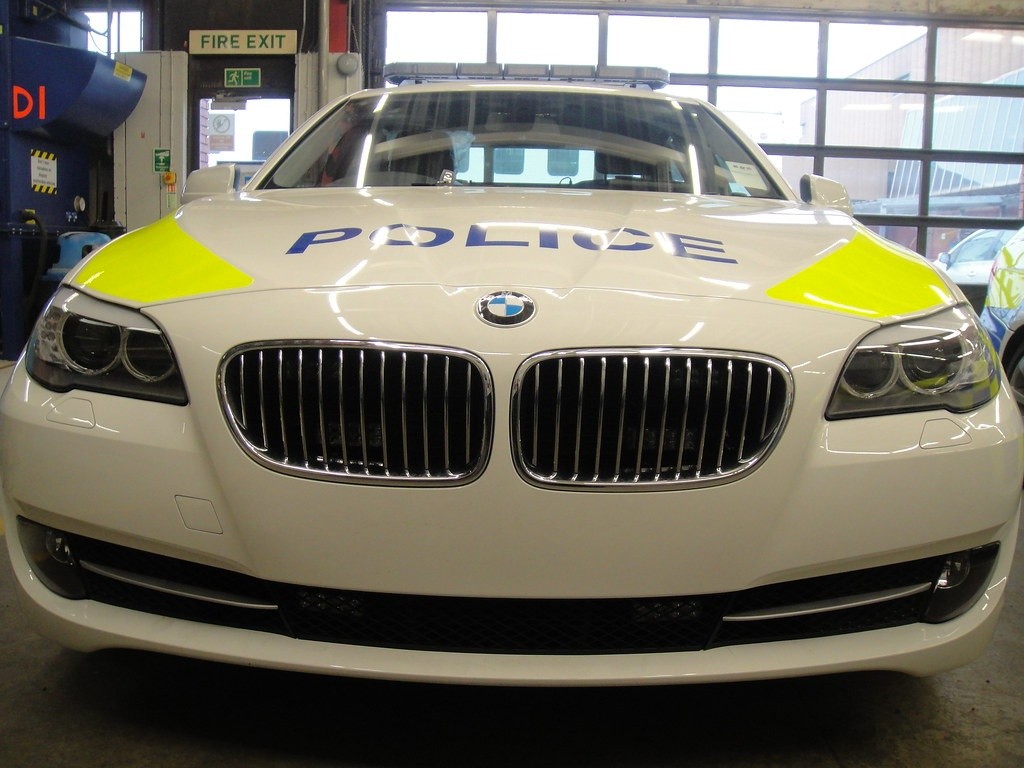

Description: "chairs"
321 125 456 187
597 125 677 192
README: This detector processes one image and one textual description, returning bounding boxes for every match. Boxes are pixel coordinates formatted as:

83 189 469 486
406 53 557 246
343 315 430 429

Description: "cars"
0 62 1024 689
975 226 1024 403
925 225 1024 302
